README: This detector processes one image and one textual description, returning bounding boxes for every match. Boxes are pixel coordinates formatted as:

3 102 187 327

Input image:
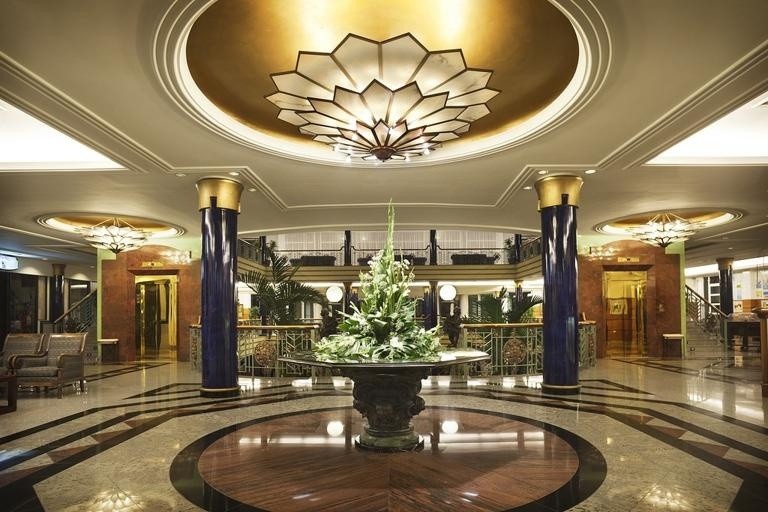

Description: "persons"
448 306 462 348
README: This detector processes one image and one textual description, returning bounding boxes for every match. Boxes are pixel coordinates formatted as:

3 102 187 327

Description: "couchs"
289 253 495 266
13 333 87 399
0 334 45 390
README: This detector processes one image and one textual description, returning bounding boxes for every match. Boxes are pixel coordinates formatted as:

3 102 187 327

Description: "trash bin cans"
662 333 684 361
102 339 120 364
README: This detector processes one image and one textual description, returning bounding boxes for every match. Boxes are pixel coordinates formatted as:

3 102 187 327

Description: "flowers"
309 197 444 361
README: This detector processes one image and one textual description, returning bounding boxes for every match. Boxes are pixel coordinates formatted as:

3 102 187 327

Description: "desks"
0 374 18 415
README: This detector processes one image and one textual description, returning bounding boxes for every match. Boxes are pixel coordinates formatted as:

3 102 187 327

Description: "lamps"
251 32 500 164
324 419 345 438
437 281 457 302
74 213 151 254
625 209 708 249
442 419 459 434
320 283 344 305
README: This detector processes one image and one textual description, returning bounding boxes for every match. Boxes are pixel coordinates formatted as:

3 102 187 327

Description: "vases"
280 344 490 451
